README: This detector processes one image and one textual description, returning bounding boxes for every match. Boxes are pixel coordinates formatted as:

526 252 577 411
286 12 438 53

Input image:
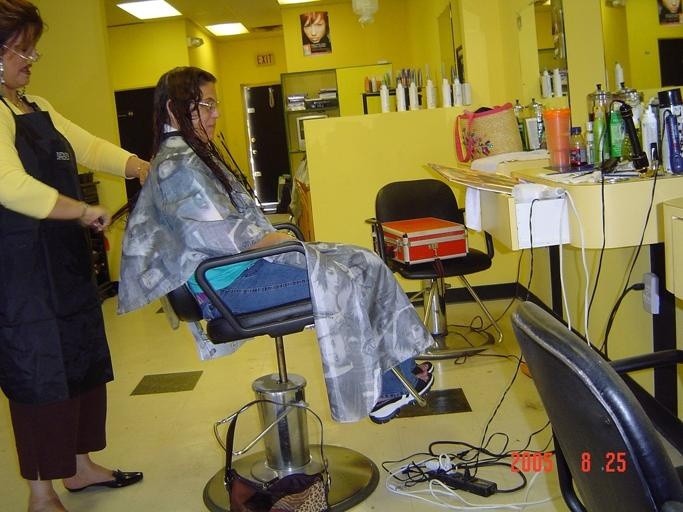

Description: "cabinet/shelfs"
80 172 119 302
280 61 397 155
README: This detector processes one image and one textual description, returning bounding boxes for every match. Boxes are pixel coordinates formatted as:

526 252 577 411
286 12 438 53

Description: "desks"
470 149 681 380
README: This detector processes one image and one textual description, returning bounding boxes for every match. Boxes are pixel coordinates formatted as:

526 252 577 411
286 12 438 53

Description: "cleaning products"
614 59 624 89
552 67 563 96
541 68 551 97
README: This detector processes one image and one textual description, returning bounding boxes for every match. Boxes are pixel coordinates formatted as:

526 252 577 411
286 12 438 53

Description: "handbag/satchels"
456 104 523 162
224 468 329 512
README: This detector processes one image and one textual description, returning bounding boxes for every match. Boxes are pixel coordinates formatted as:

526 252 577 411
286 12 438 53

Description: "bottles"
363 66 471 113
540 68 562 98
513 97 543 149
615 64 625 90
542 82 683 178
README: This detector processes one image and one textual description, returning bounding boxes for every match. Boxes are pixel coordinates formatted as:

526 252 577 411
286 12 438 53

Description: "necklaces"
13 93 23 108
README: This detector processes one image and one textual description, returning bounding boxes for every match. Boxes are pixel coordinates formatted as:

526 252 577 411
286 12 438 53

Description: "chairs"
125 188 383 510
364 178 502 361
510 298 683 511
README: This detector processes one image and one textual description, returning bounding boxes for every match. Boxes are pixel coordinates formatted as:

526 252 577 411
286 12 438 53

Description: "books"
286 87 338 112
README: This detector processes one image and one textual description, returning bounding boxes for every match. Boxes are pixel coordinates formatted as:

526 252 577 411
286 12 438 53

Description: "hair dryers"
509 181 567 200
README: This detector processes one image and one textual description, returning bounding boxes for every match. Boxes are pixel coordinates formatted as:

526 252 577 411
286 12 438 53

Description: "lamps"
352 0 381 28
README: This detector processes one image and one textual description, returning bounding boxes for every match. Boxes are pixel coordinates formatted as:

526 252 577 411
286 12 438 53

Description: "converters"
425 460 440 471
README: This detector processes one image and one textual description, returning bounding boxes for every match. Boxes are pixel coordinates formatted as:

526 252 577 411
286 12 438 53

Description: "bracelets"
136 160 141 177
80 202 87 220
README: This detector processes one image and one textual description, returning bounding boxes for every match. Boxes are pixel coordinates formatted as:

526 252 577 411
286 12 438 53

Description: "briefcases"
381 217 469 267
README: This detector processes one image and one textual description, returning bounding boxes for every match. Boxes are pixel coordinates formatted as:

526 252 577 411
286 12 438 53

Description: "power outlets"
640 273 661 315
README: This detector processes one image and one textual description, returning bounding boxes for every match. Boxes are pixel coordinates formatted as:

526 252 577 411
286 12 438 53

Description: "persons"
0 2 143 512
656 0 683 27
149 66 435 426
299 11 332 57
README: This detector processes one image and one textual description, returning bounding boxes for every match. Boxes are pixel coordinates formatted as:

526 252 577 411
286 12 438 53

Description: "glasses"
1 44 42 64
195 100 223 111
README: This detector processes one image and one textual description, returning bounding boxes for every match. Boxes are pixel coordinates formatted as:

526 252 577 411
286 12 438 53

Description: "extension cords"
423 467 497 498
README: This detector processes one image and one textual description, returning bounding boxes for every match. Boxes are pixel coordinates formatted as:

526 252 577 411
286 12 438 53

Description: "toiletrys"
364 66 472 113
582 121 596 165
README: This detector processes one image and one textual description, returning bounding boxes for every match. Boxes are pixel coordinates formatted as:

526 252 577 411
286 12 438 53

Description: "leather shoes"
64 471 143 491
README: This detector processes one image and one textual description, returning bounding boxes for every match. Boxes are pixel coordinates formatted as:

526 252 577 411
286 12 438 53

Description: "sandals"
368 361 434 425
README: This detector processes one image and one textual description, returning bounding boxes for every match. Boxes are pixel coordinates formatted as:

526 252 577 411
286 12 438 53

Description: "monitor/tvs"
295 114 329 151
